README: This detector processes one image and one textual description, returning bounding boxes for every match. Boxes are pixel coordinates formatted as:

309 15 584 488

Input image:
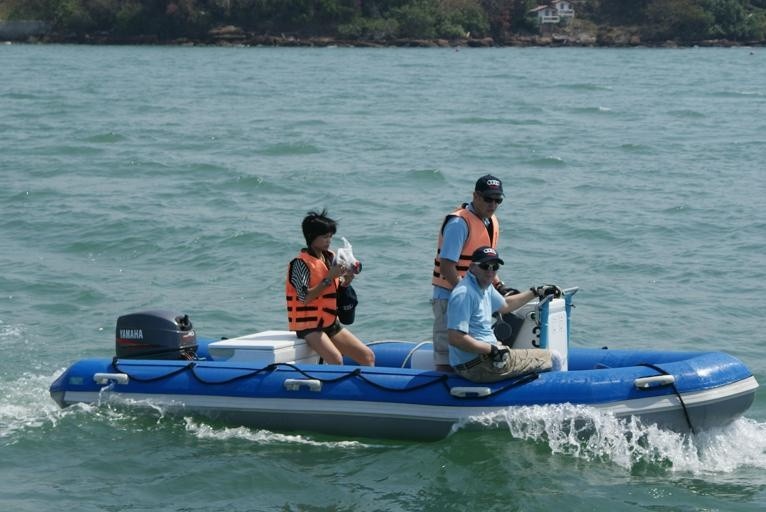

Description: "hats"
336 284 358 325
472 247 504 265
475 175 505 200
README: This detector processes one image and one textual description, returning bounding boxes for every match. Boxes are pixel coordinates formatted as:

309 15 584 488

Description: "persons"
447 246 566 384
432 173 506 371
285 208 375 366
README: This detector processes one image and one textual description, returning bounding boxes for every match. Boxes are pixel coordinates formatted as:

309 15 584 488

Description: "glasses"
477 261 499 271
477 193 503 204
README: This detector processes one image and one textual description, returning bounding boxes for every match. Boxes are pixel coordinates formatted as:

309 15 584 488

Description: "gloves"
488 344 512 369
530 283 562 302
494 280 521 297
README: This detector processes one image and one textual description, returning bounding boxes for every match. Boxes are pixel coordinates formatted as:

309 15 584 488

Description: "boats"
47 333 759 444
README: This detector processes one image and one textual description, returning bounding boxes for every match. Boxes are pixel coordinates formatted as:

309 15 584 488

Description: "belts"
455 353 489 371
